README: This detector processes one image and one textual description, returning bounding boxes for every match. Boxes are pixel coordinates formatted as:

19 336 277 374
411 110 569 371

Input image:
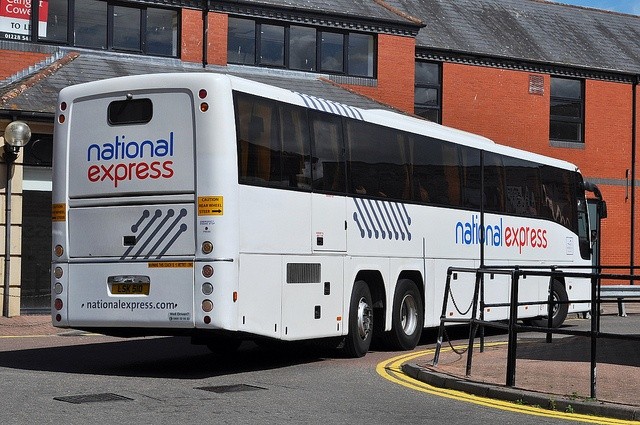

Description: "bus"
50 71 608 357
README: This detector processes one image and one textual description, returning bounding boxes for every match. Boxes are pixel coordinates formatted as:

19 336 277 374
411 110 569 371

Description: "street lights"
2 120 31 316
